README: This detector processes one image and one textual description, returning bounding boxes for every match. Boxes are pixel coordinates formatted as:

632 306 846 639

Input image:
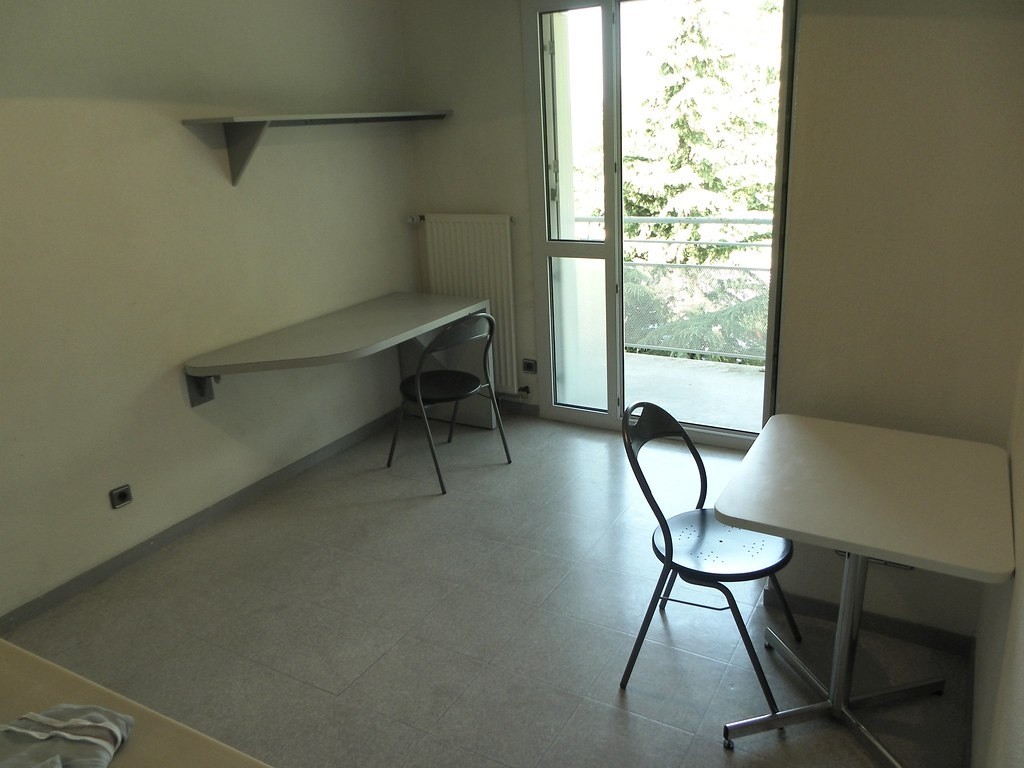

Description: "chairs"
388 311 512 495
621 402 796 738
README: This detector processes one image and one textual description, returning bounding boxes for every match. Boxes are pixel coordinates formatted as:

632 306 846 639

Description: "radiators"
404 214 527 394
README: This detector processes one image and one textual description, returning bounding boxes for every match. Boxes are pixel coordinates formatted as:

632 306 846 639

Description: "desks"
715 414 1016 768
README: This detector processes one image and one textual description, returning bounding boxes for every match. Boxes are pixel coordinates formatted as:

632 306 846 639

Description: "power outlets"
522 359 536 375
110 484 133 509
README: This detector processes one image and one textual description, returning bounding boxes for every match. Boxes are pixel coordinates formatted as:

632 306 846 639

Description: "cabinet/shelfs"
180 109 499 378
